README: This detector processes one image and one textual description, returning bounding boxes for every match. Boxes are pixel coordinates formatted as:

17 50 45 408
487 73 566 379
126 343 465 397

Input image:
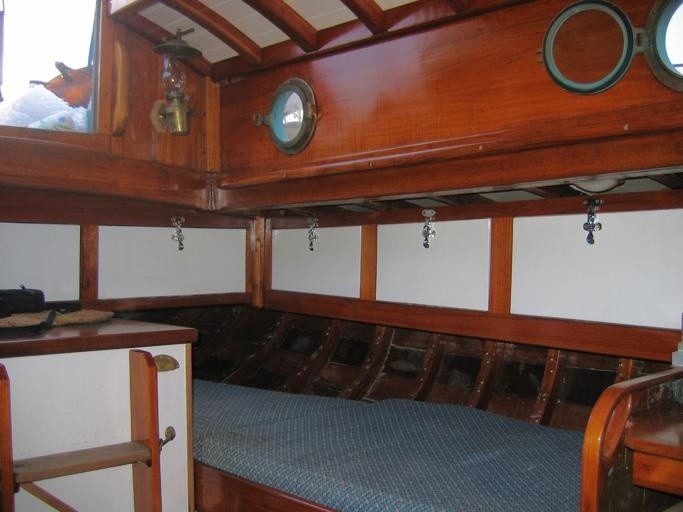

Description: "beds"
100 291 683 510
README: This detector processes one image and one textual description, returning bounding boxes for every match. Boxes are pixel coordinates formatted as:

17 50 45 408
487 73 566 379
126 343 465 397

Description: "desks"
623 399 683 497
0 318 197 511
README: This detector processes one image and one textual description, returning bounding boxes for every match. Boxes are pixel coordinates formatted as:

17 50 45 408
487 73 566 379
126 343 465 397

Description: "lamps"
152 27 202 137
